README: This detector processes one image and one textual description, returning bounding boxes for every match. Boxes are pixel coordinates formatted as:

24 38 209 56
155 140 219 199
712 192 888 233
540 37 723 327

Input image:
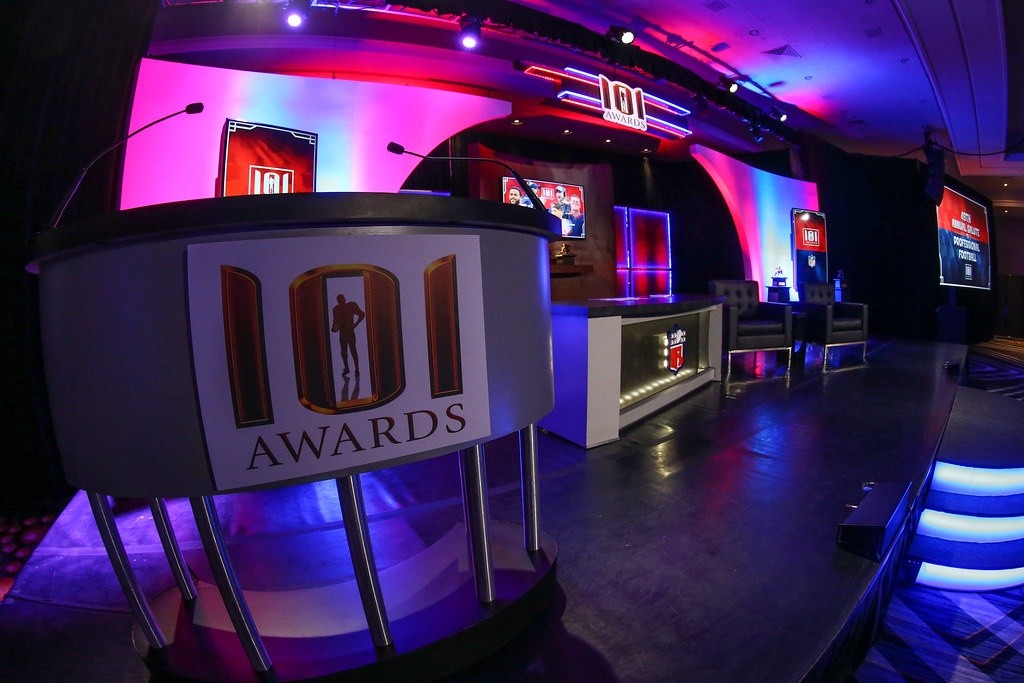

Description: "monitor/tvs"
502 175 586 239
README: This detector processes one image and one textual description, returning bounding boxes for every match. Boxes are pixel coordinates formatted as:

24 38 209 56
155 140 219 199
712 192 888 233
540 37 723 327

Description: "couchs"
794 282 869 360
707 280 794 373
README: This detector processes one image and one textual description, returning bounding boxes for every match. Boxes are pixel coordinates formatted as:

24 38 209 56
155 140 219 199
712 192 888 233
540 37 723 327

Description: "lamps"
695 97 710 117
281 0 310 30
748 123 764 144
604 25 636 46
768 105 787 122
460 21 483 48
714 75 738 93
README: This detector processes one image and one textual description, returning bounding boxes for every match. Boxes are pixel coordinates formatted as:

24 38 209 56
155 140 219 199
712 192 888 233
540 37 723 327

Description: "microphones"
386 142 549 214
47 101 204 230
